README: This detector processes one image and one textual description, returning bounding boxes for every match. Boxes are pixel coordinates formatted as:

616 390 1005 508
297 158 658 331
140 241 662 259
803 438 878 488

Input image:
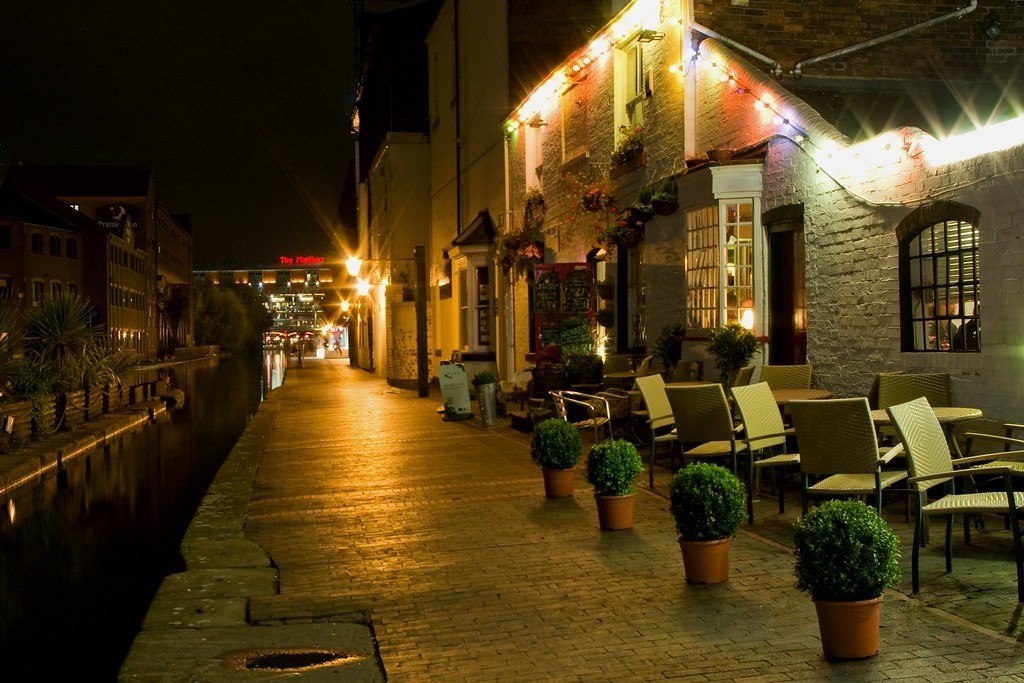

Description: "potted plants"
471 370 498 426
627 184 656 222
649 175 679 215
668 460 750 583
528 418 584 499
581 436 646 530
0 288 131 453
791 497 904 661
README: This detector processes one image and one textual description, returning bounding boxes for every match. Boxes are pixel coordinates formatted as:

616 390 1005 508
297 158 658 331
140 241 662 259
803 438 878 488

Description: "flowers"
516 243 543 260
617 124 644 137
559 174 625 242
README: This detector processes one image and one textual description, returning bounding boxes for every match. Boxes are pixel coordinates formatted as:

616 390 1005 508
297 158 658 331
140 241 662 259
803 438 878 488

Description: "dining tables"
727 388 832 496
608 369 667 378
869 405 987 532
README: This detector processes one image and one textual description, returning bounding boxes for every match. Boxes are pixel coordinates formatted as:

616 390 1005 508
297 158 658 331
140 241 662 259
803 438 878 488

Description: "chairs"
634 373 685 488
885 395 1024 603
874 372 966 478
730 381 802 524
759 364 813 390
548 389 614 447
959 423 1024 531
594 387 646 449
664 382 749 478
